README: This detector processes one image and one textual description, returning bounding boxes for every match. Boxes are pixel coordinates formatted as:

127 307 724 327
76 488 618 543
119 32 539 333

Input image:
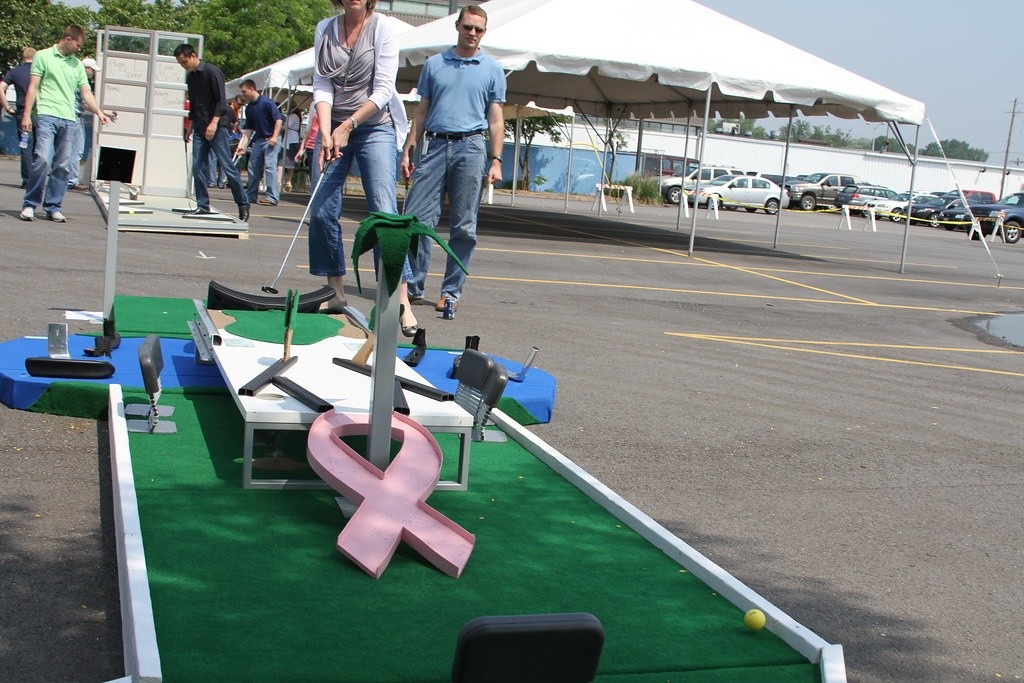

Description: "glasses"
461 21 485 34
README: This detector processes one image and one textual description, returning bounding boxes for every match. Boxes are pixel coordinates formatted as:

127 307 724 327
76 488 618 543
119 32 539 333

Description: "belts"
427 128 480 140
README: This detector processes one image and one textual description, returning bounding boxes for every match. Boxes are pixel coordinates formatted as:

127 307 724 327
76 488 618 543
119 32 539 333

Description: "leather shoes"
238 202 250 222
190 208 209 214
401 322 418 337
408 295 424 305
436 297 457 312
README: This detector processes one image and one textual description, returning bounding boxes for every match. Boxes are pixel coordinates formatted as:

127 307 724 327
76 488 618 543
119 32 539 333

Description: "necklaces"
343 9 368 48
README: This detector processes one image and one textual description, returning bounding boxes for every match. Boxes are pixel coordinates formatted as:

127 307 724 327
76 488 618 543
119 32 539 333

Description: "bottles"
20 131 29 149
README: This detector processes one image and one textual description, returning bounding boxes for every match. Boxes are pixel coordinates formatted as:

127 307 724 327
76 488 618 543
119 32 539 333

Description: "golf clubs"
182 140 193 216
260 146 336 295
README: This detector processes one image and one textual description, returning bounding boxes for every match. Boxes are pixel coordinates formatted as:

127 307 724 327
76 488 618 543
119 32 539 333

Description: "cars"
639 150 1024 245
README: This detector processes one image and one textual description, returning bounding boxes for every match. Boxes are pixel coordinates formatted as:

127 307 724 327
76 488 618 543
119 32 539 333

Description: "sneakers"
45 211 66 222
20 207 34 221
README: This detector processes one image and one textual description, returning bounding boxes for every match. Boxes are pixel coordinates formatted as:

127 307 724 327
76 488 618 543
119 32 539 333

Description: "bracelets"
6 107 11 113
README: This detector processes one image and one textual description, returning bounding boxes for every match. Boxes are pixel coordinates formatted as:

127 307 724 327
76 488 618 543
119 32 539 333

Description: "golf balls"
743 609 766 631
128 209 135 215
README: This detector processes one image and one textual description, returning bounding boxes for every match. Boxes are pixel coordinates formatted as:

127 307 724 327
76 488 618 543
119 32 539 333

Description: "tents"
224 0 925 274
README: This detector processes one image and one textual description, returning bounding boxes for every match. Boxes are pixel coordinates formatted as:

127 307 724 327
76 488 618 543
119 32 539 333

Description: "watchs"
490 155 503 163
349 116 358 128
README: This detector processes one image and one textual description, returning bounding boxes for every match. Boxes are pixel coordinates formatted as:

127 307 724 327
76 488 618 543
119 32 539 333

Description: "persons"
0 24 117 221
174 44 250 223
310 0 418 335
399 5 507 313
208 79 319 207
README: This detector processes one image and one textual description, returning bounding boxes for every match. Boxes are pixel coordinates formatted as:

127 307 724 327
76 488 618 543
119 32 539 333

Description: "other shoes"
70 183 88 191
259 199 276 204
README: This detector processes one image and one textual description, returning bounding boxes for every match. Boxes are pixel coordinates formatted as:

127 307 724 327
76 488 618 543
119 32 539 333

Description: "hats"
82 58 101 72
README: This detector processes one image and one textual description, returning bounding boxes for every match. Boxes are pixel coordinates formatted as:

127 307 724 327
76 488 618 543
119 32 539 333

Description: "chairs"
878 193 883 197
